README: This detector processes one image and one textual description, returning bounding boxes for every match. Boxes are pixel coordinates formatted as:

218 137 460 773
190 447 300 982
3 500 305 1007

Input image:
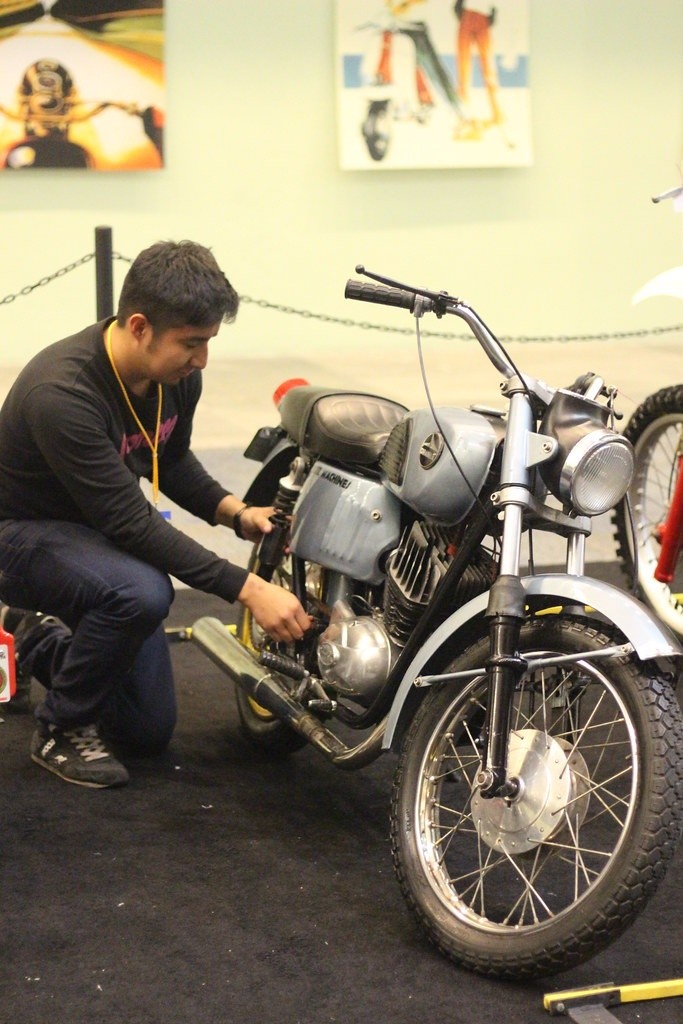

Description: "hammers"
165 623 237 640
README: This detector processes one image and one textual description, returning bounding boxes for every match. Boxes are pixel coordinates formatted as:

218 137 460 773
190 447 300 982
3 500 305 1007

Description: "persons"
386 1 505 126
0 241 314 788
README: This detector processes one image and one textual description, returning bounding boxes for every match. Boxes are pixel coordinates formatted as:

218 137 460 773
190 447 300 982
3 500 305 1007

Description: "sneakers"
31 721 130 790
1 603 38 711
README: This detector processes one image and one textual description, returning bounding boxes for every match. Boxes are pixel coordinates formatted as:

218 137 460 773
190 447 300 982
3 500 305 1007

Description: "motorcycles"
184 264 683 982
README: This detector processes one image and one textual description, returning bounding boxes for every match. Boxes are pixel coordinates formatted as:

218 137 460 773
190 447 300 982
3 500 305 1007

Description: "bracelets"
234 506 250 540
